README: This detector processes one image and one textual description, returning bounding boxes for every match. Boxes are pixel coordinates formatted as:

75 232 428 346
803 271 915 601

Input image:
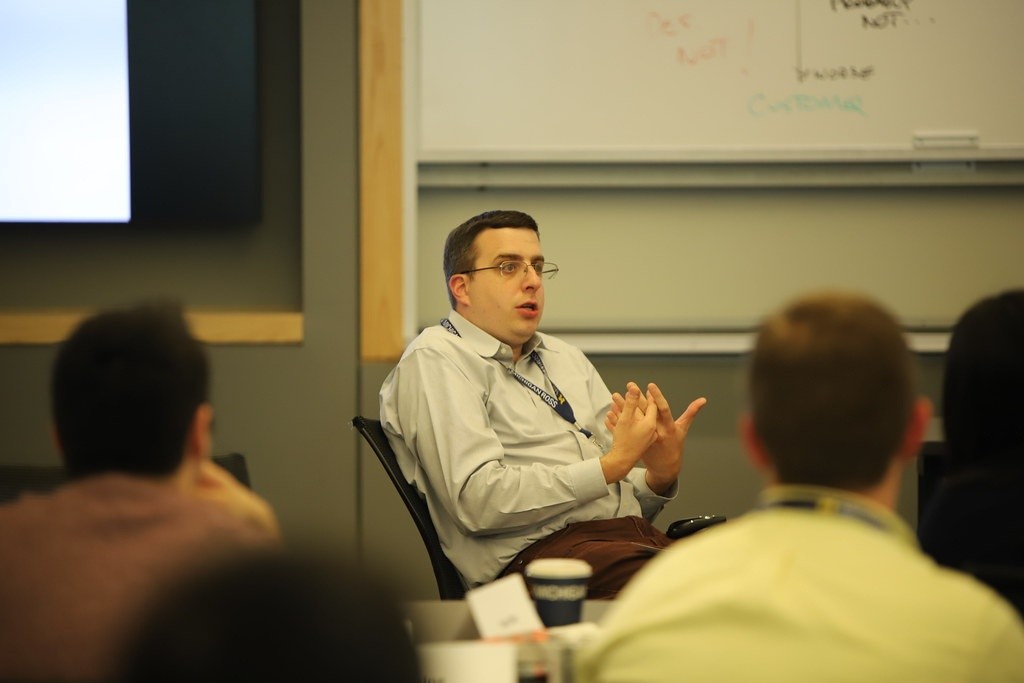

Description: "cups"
526 557 593 628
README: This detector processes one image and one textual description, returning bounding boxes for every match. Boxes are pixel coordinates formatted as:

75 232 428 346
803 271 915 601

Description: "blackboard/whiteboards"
400 0 1023 354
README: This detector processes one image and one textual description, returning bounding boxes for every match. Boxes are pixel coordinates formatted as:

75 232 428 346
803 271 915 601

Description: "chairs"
352 415 724 603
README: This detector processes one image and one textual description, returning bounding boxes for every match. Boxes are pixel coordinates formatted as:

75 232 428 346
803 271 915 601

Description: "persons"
379 209 707 600
594 292 1024 683
0 305 423 683
928 288 1024 614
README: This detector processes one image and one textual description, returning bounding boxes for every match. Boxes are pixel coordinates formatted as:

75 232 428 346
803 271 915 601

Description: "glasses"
459 261 559 281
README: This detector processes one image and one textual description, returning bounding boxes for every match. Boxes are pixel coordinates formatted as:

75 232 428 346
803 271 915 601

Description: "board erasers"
913 131 979 150
